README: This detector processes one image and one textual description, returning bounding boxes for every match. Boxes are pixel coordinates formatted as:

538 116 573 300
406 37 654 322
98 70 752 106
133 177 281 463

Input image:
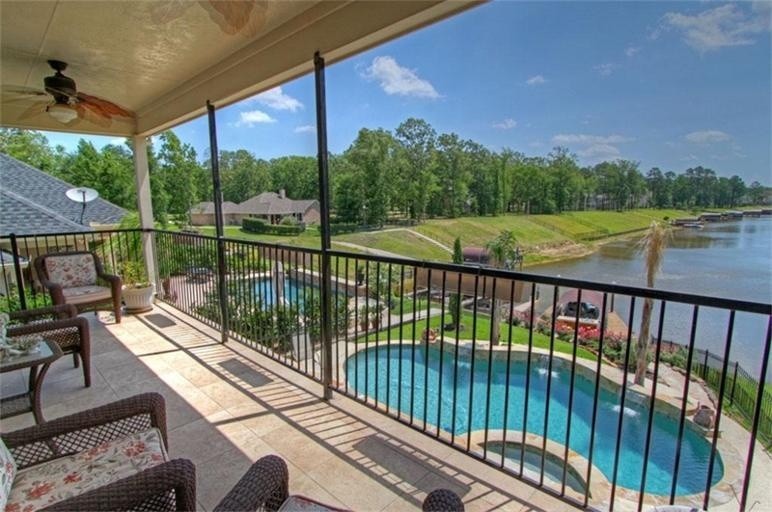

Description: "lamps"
37 59 84 125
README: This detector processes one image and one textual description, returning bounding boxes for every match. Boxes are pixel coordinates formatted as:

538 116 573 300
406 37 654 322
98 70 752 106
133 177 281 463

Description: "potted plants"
116 258 160 315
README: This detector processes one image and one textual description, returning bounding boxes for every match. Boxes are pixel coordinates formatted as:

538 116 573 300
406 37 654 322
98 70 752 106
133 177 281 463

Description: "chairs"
1 303 98 387
33 249 124 327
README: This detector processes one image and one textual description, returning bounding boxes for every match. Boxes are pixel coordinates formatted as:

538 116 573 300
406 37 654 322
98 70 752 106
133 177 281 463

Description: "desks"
0 337 76 455
